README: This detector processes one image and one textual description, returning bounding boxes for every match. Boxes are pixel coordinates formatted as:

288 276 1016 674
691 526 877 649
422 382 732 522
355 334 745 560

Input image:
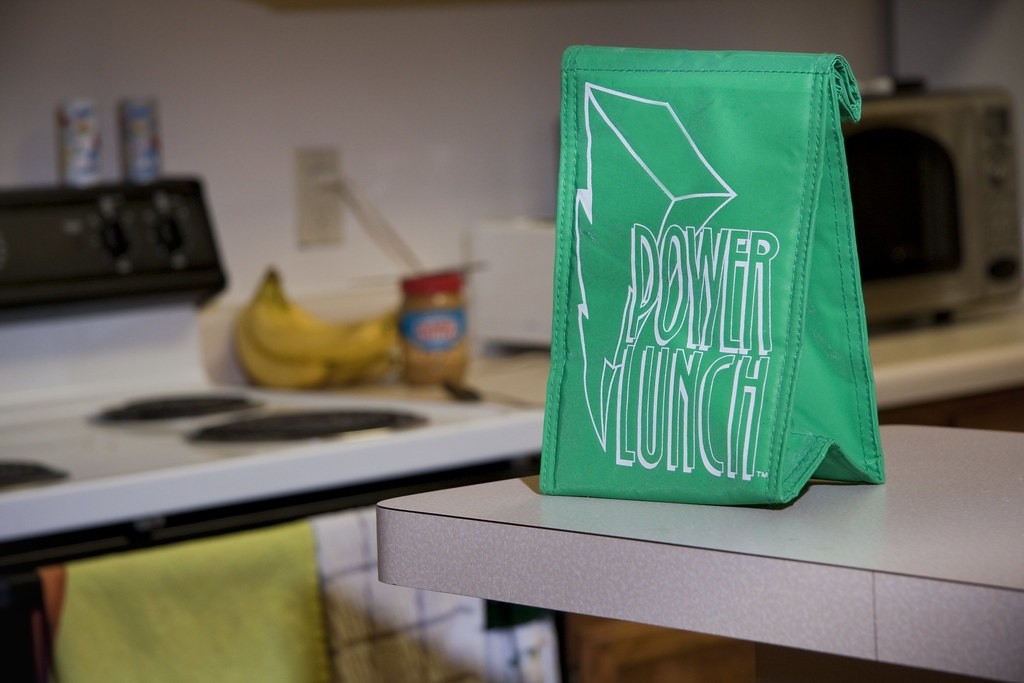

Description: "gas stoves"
0 176 542 539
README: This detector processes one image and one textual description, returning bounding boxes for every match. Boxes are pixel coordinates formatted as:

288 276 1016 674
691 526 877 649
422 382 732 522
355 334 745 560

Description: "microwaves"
840 87 1024 333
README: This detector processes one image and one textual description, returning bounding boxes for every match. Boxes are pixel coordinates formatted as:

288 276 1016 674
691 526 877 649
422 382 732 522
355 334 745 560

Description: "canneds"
400 270 469 388
118 96 163 182
56 97 101 184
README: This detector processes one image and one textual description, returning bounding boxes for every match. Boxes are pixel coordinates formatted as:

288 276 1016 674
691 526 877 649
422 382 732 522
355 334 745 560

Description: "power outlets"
292 146 344 249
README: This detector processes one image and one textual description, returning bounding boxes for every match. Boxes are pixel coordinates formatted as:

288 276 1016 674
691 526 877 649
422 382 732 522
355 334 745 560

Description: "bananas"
236 271 402 390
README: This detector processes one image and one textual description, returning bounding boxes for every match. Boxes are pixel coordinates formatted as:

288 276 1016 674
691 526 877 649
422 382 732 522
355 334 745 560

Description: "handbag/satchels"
539 45 886 506
